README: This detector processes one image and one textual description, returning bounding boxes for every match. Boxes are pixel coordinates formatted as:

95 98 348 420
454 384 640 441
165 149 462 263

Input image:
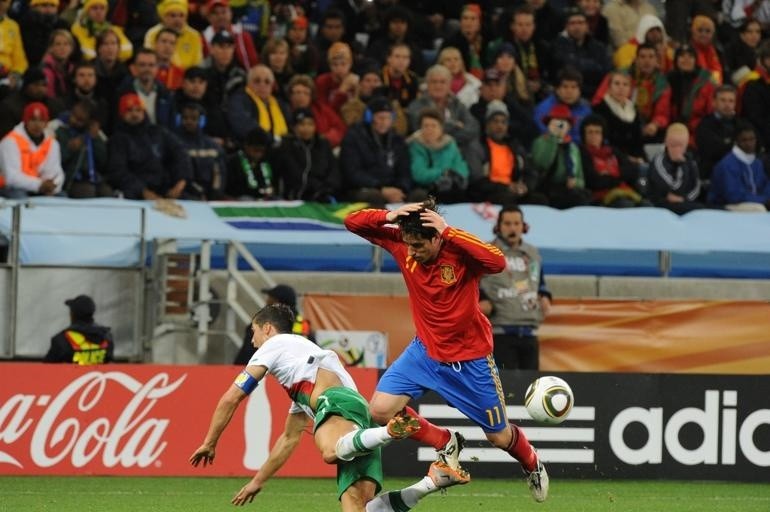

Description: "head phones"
493 207 528 234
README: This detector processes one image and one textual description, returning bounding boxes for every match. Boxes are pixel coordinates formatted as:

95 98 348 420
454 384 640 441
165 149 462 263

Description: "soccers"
525 376 574 427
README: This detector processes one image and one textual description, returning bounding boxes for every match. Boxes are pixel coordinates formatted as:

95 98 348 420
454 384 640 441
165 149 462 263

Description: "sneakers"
434 428 466 471
428 459 473 488
519 444 550 503
384 413 421 440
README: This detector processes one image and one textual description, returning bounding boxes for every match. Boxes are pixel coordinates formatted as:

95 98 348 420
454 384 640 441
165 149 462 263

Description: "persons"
536 69 593 142
343 58 405 131
202 1 259 72
155 29 184 90
188 302 471 512
21 0 83 67
71 0 133 62
261 1 309 47
44 295 113 365
225 65 306 199
405 63 487 202
233 284 318 366
0 68 62 140
56 98 117 197
521 1 770 28
53 64 109 130
480 206 554 371
171 101 226 199
201 30 246 98
530 104 586 205
38 30 79 99
284 108 342 201
384 42 413 105
107 92 194 200
649 122 702 203
482 39 537 148
593 43 672 137
666 43 717 133
88 28 127 98
310 42 359 148
735 51 770 118
142 0 204 68
171 67 229 149
280 73 315 122
724 19 763 83
404 111 470 202
579 114 653 207
674 16 723 85
417 44 483 108
708 120 770 211
695 85 770 167
361 8 425 75
552 15 613 97
260 37 297 99
483 99 529 203
488 8 554 102
0 1 29 99
595 73 646 159
336 98 412 202
438 4 486 81
111 49 178 124
0 101 69 198
298 12 364 76
611 15 673 72
344 197 549 502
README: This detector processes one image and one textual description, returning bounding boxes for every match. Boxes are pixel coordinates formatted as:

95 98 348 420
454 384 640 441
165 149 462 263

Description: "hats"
485 98 510 121
539 103 581 129
261 282 299 311
79 0 108 13
64 293 96 324
157 0 187 15
117 93 145 118
288 105 315 123
21 101 49 127
367 99 394 113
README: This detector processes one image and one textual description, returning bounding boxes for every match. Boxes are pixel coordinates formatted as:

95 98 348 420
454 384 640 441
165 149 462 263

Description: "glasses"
252 75 273 85
